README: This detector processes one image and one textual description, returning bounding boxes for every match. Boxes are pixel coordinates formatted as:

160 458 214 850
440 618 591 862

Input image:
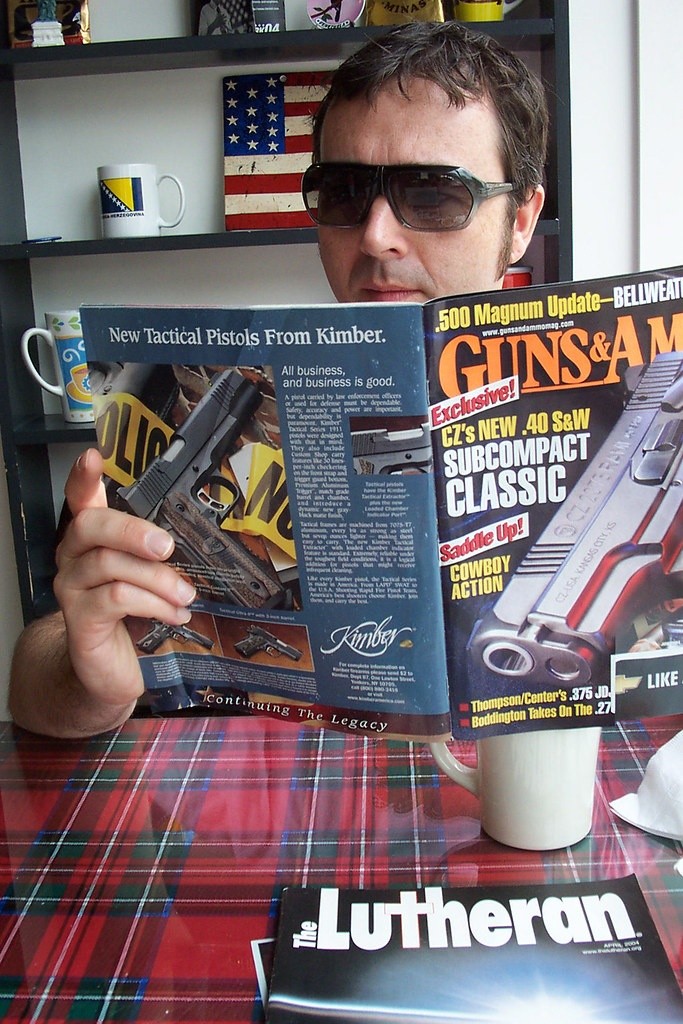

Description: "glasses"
301 162 520 234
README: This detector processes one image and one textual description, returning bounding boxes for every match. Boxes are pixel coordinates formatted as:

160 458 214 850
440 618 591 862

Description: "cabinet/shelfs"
0 0 574 628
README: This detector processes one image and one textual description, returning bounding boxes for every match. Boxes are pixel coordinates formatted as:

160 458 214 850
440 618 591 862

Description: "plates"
306 0 365 29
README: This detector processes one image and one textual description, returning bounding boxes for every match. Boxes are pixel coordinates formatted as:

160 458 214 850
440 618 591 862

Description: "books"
262 873 683 1023
79 265 682 739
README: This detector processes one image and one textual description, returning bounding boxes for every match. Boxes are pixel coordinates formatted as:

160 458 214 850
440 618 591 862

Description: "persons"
6 18 550 740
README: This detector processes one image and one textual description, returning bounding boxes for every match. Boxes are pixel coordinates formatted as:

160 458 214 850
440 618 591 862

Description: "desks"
0 713 683 1024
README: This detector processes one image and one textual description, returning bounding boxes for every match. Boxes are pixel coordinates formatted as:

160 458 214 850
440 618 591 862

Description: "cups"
96 164 186 238
428 726 601 851
20 311 94 423
452 0 504 22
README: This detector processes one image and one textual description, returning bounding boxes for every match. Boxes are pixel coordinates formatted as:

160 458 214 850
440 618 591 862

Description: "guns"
108 370 291 610
467 345 683 687
138 622 215 654
234 624 303 662
